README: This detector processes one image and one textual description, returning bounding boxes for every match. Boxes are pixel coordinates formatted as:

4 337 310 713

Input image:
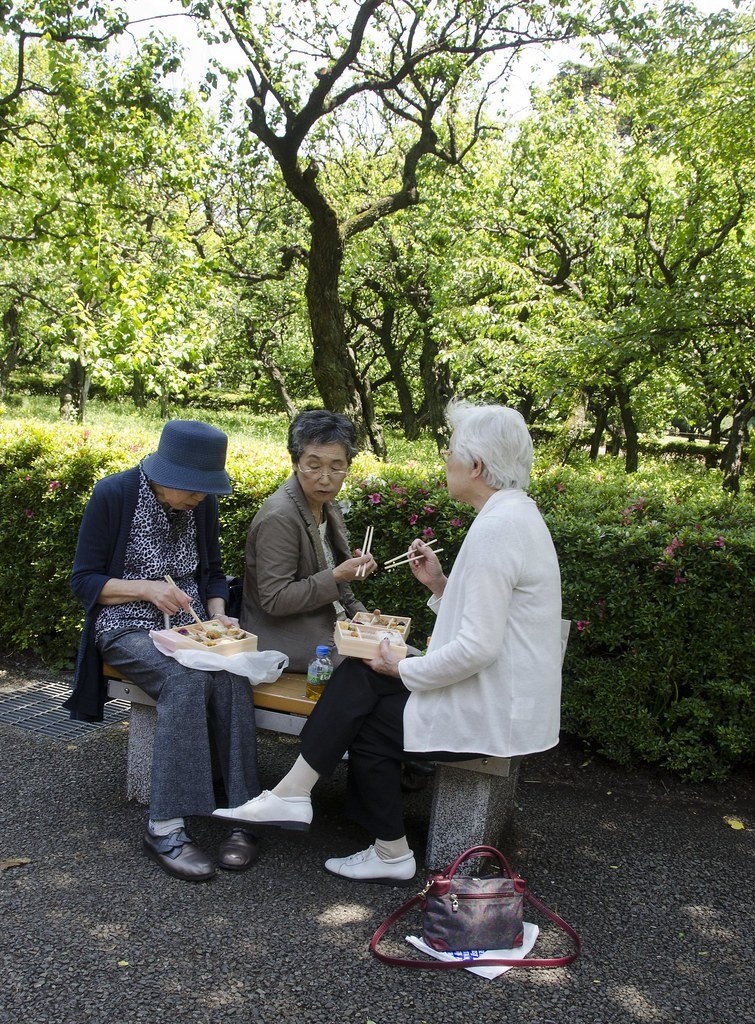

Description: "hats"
144 421 232 493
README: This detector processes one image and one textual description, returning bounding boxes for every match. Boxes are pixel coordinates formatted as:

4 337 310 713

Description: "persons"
62 420 260 880
209 402 565 882
238 404 435 791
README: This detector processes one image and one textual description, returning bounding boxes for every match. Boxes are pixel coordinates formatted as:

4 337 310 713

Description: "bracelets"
211 614 223 617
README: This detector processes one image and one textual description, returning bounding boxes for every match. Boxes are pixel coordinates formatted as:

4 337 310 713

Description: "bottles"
305 645 333 702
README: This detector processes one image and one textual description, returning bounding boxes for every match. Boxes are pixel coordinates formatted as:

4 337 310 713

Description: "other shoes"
400 771 428 792
405 760 436 775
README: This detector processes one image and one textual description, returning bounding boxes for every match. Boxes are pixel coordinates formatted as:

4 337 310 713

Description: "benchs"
103 660 522 878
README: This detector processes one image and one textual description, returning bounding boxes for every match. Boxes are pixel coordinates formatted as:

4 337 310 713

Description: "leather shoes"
142 826 215 881
324 845 416 887
212 789 313 829
217 825 259 870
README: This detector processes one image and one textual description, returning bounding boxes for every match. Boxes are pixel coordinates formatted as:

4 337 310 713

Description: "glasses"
296 462 349 482
439 449 457 461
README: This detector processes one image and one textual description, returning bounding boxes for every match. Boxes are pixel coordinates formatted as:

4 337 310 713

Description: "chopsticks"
356 526 374 577
384 539 444 570
163 575 207 632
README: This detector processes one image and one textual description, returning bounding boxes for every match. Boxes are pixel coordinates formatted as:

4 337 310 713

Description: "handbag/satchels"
371 845 581 970
